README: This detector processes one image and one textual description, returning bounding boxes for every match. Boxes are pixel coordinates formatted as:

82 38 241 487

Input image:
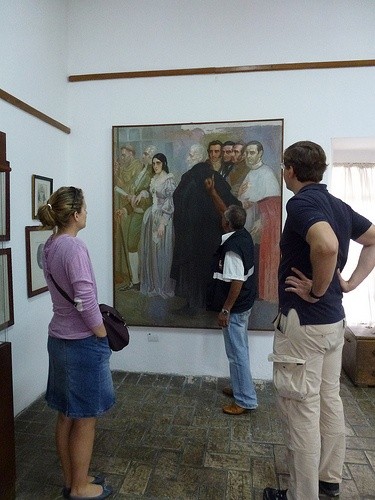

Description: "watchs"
222 308 229 316
309 288 326 299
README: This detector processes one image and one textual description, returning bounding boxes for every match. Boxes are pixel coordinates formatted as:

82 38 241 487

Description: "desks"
343 323 375 387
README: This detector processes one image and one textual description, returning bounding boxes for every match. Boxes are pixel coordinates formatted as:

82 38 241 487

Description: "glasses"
279 162 290 169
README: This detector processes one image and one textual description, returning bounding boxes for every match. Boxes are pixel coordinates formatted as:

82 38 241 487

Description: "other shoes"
222 383 234 397
68 483 114 500
63 475 105 497
223 403 248 415
263 486 291 500
319 479 342 497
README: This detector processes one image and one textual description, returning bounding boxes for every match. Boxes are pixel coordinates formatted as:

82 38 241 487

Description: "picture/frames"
0 248 14 332
26 225 54 298
32 175 53 220
112 118 285 331
0 161 10 241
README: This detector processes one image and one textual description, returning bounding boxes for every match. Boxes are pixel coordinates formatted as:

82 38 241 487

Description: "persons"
261 141 375 500
204 173 258 415
36 185 117 500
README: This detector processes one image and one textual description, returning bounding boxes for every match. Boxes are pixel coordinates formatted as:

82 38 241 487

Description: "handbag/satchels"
98 304 131 353
